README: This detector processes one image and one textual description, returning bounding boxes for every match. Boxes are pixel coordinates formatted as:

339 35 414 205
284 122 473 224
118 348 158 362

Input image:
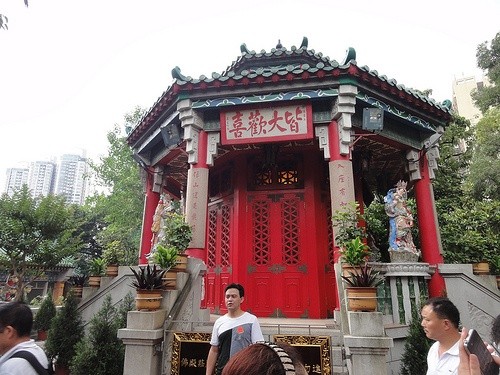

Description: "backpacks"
8 350 69 375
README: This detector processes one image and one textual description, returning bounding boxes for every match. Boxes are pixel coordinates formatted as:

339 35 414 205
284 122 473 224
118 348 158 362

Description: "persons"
143 192 175 257
205 283 310 375
384 180 418 253
0 301 52 375
420 298 500 375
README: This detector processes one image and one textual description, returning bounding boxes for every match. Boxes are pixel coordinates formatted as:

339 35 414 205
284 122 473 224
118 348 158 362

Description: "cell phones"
464 329 500 375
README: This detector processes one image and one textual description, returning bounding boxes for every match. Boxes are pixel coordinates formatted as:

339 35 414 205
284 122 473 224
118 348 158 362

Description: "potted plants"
340 262 385 312
68 273 89 297
166 214 193 270
86 257 107 287
339 235 375 281
125 264 172 311
153 245 181 289
105 247 120 277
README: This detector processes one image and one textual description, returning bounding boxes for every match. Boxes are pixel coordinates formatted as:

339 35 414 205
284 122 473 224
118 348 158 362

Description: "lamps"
160 124 189 159
129 154 154 176
351 106 385 146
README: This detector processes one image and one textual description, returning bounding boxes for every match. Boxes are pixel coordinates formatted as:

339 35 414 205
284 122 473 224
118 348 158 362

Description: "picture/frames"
171 332 218 375
273 334 333 375
